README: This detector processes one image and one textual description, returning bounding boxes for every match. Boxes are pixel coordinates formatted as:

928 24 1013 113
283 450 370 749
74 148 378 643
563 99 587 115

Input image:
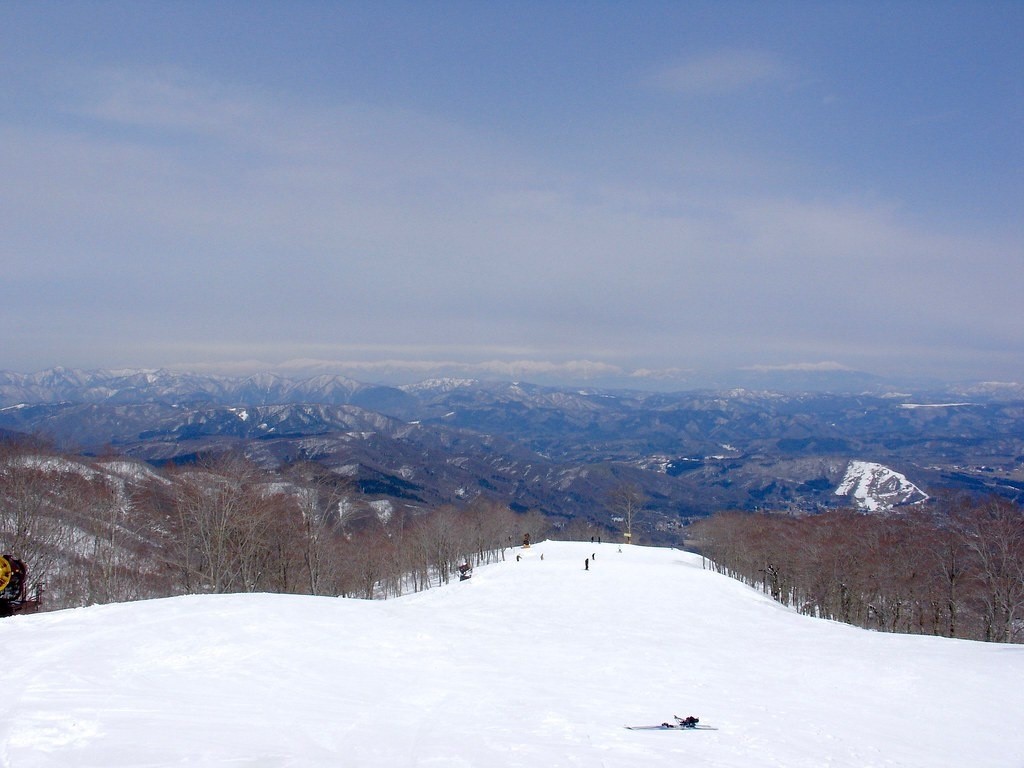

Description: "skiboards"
622 714 720 731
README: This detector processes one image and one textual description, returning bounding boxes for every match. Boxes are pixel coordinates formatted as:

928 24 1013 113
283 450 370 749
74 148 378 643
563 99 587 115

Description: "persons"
617 545 622 553
585 559 589 570
592 553 596 560
591 536 594 543
598 536 601 543
517 554 522 561
541 554 544 560
